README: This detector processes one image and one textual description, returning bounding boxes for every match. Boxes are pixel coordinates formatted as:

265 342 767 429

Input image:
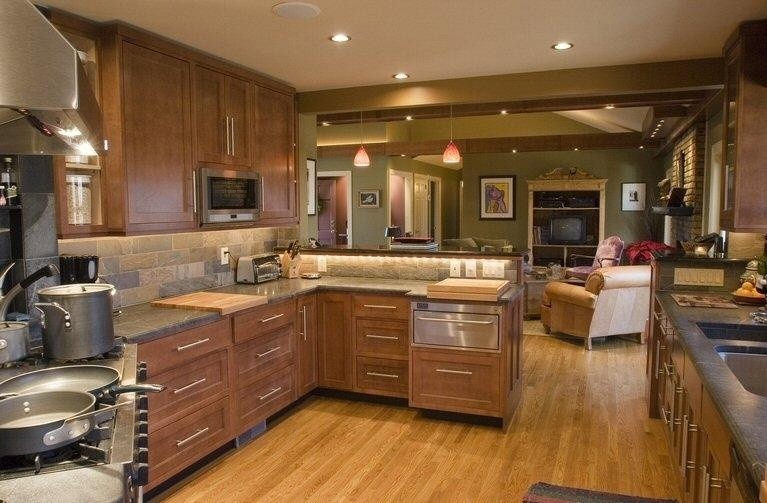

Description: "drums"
288 239 301 259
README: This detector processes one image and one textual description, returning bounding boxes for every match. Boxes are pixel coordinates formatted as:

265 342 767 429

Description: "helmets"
730 293 766 304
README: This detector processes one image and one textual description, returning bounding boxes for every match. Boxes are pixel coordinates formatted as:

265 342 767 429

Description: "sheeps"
749 312 767 324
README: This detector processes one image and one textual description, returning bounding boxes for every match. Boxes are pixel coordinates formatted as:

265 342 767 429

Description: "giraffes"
2 343 151 503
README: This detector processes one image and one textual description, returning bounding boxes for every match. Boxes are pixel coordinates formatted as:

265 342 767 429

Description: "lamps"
566 234 625 278
536 262 651 351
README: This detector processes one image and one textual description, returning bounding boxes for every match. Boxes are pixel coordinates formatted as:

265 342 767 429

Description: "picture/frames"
520 265 566 321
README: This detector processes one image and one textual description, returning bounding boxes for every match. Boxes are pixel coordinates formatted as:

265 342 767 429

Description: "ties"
547 214 587 245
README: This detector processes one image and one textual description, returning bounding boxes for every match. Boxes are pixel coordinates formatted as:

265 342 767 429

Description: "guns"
752 287 762 297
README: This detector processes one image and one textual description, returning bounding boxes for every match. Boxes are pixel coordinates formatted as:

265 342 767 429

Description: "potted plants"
306 158 317 215
478 174 517 220
620 181 648 212
355 188 380 209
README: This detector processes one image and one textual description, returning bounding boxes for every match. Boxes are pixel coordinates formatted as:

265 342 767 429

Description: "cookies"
198 166 260 222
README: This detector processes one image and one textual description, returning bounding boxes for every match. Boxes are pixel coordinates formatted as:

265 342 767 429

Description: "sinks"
442 235 513 252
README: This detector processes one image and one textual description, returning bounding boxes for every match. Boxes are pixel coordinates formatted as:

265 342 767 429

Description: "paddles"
521 479 681 502
519 319 609 341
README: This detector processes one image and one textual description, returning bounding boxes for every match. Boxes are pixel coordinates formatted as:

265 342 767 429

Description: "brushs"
0 0 107 158
60 252 100 285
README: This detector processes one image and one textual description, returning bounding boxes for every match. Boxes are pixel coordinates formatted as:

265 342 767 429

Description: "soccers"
737 282 753 295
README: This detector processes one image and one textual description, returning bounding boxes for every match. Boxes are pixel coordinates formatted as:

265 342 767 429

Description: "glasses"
443 104 460 162
354 111 371 166
384 223 402 239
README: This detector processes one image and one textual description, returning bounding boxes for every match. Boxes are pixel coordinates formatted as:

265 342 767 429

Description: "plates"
184 46 253 171
98 23 195 237
244 70 303 227
648 305 759 502
136 316 233 493
526 166 608 267
405 292 530 433
292 284 317 402
347 294 408 402
228 296 297 445
317 291 354 395
720 17 767 233
42 6 106 240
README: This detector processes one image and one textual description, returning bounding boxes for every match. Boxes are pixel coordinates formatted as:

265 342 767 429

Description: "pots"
0 365 168 457
0 321 30 362
34 282 118 360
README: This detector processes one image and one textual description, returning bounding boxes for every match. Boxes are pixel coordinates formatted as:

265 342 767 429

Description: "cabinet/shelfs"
65 171 92 225
1 158 21 208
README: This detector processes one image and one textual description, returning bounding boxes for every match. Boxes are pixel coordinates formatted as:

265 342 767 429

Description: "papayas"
236 252 282 284
